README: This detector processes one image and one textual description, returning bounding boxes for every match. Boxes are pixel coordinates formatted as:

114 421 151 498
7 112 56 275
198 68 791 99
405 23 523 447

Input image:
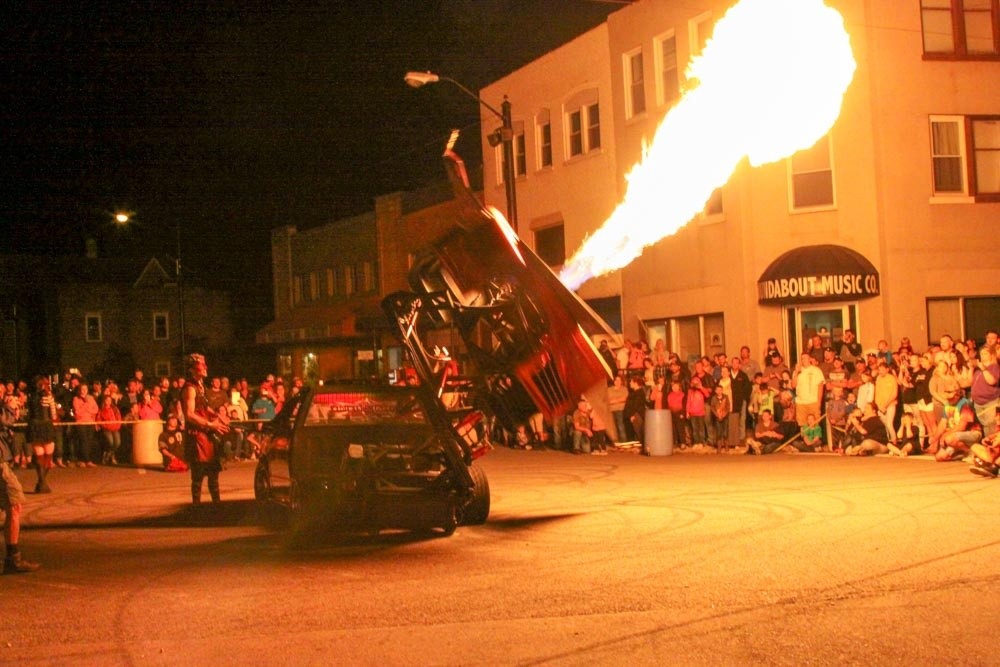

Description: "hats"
945 382 961 398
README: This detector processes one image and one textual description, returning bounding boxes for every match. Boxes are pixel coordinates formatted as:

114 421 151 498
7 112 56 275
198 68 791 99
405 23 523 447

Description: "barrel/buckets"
642 409 672 456
132 422 164 465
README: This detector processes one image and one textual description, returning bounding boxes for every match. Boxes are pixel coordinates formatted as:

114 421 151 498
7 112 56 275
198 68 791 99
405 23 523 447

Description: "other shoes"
971 466 999 477
35 483 52 493
14 460 98 468
5 552 40 572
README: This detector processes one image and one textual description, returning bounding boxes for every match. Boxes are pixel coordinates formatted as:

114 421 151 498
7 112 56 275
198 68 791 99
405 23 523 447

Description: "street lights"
115 212 188 380
404 71 518 236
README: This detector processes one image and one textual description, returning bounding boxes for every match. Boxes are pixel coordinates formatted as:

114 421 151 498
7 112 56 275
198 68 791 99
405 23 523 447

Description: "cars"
253 372 491 538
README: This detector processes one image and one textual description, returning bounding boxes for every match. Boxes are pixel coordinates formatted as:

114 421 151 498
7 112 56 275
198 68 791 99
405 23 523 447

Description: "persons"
451 325 1000 479
180 354 230 512
0 402 42 575
0 370 187 468
406 376 418 417
205 375 320 462
24 375 57 494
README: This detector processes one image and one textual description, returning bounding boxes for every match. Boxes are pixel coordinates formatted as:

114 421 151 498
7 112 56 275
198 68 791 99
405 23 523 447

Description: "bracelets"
206 422 212 429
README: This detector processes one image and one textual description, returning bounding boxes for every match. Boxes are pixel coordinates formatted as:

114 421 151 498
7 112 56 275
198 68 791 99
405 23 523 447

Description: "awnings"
758 245 880 304
